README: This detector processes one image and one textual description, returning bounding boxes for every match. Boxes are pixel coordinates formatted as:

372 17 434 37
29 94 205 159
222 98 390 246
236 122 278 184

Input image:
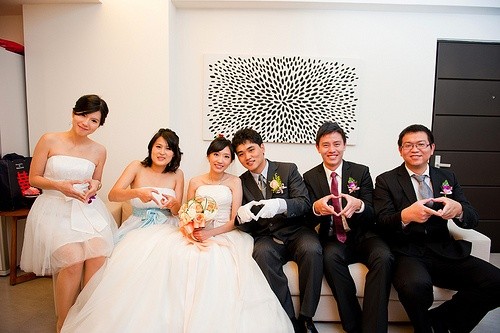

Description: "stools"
1 209 39 286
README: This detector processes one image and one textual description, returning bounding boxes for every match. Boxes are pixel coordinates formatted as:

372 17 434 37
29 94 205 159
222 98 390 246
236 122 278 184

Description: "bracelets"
98 182 102 191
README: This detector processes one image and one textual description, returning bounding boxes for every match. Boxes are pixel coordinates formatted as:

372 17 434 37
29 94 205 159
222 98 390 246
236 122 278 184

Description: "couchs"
52 199 491 322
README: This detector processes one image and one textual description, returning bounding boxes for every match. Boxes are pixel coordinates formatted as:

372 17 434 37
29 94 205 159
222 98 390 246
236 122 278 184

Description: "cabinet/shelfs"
429 36 500 254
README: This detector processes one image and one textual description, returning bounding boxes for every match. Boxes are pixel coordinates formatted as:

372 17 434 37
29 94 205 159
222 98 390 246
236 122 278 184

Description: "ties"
329 172 347 244
257 175 266 201
413 175 434 207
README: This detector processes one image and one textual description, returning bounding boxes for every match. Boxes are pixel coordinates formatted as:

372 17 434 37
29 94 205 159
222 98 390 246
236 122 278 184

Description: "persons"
19 95 118 333
303 122 395 333
232 130 324 333
62 136 295 333
375 124 500 333
109 127 185 235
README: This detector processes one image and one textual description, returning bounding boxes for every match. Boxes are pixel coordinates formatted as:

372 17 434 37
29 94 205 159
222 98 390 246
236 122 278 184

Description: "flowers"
268 172 288 194
438 180 452 196
346 175 360 193
177 196 219 231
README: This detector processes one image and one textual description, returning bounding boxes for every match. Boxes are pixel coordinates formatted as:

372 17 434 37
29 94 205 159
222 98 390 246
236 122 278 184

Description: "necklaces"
209 173 224 185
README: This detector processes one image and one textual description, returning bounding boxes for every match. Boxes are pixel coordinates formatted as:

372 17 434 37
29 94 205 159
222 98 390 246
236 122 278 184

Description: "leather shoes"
290 318 302 332
298 314 318 333
